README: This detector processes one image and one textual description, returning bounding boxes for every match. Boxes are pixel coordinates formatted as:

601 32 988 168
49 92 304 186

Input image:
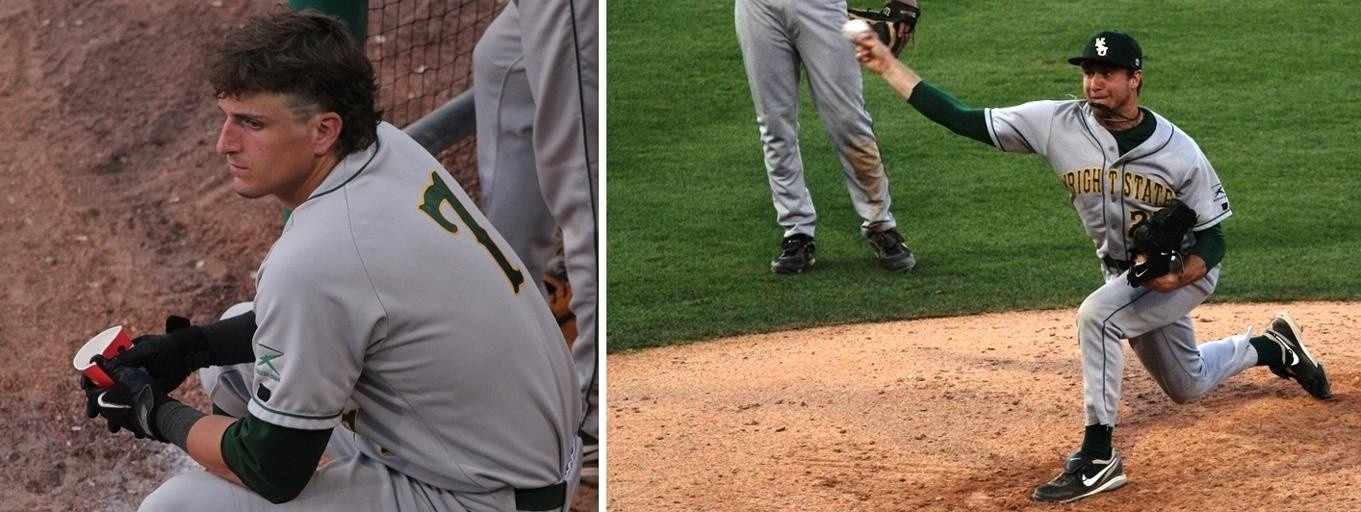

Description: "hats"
1068 32 1144 72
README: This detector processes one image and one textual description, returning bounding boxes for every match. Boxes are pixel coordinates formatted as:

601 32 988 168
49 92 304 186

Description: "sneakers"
1032 448 1128 503
1265 311 1330 399
770 235 819 275
866 226 918 272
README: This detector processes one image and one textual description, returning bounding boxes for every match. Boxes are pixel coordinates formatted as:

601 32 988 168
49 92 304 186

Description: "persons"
841 18 1334 503
82 6 587 512
733 0 917 277
469 1 599 491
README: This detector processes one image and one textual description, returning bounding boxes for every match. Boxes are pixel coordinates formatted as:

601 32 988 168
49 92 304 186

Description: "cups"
73 325 136 390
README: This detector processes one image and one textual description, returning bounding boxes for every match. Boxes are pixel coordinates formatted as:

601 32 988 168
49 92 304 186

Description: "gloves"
78 314 196 445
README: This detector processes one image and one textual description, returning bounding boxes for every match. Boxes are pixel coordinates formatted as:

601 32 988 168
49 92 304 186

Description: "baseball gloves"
1127 198 1199 287
847 0 921 57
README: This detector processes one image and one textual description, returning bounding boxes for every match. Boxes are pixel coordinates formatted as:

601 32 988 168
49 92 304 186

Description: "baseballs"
841 19 871 43
